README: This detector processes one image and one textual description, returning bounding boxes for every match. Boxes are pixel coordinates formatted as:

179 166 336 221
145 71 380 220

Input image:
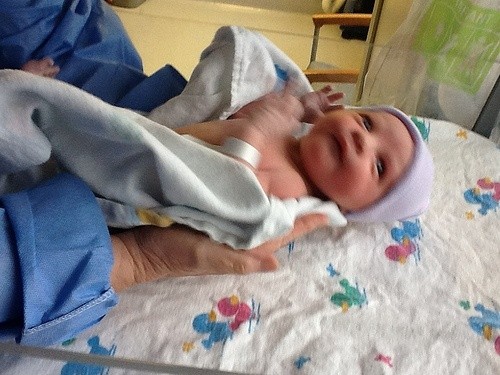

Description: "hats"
343 104 436 223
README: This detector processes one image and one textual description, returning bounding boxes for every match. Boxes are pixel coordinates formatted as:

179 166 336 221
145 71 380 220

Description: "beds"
1 118 500 375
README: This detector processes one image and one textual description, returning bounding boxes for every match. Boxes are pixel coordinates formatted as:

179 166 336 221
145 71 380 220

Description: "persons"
0 0 330 347
2 56 439 233
